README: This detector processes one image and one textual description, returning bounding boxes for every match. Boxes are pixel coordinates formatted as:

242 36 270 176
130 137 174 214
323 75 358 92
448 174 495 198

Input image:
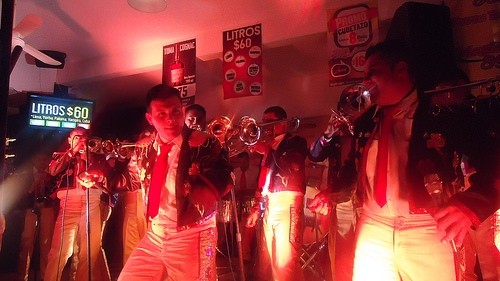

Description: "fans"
11 0 62 66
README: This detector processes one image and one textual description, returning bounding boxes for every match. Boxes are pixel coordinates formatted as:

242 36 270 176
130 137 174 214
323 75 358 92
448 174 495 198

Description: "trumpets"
113 138 143 160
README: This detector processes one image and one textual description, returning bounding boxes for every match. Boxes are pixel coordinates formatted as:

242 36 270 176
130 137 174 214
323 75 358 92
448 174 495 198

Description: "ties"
257 138 275 188
79 159 87 191
147 144 173 219
372 109 397 209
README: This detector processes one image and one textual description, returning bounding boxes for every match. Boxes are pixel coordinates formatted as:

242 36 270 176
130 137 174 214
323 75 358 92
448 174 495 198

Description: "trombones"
238 114 305 145
330 83 372 135
196 114 234 144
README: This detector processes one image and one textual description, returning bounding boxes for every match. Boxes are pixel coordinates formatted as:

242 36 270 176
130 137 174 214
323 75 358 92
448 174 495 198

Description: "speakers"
382 2 455 55
35 50 67 68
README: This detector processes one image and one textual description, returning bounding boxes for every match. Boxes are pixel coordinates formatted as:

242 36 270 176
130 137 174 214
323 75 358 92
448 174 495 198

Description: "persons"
233 151 268 281
76 84 236 281
308 101 373 281
309 41 500 281
246 106 308 281
44 126 110 281
19 150 55 281
184 104 205 132
121 128 152 268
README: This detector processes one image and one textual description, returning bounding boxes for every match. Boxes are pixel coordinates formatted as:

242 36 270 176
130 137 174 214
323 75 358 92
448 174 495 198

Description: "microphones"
424 173 461 253
75 134 92 139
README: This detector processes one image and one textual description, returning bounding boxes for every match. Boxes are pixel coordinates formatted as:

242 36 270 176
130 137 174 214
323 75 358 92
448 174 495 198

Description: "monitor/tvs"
25 93 96 131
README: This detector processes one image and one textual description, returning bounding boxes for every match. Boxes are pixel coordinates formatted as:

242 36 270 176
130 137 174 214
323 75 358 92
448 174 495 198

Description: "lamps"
127 0 167 13
